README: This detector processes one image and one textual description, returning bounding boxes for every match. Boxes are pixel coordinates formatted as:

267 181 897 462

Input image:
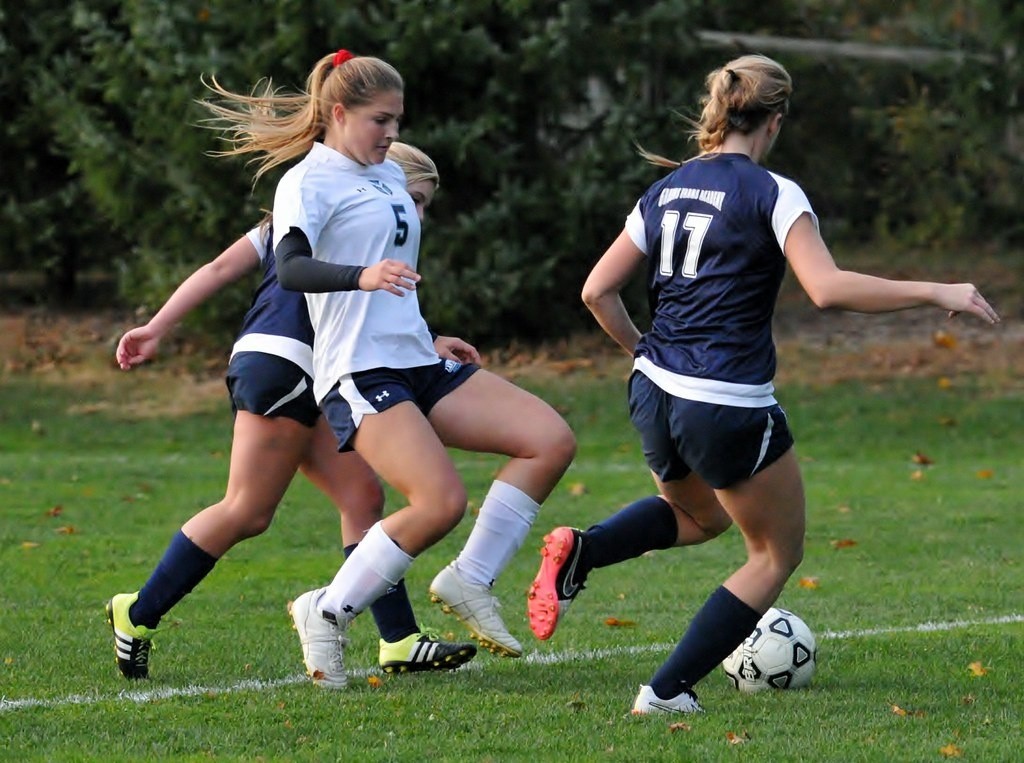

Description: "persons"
526 55 999 713
105 142 478 680
201 50 578 690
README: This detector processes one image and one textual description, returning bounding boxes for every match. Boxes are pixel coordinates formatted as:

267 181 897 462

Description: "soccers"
722 607 818 694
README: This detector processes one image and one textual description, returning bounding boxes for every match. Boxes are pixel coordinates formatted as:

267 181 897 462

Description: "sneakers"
377 625 480 673
106 590 161 680
526 525 595 642
630 682 708 721
287 585 351 692
429 558 526 659
